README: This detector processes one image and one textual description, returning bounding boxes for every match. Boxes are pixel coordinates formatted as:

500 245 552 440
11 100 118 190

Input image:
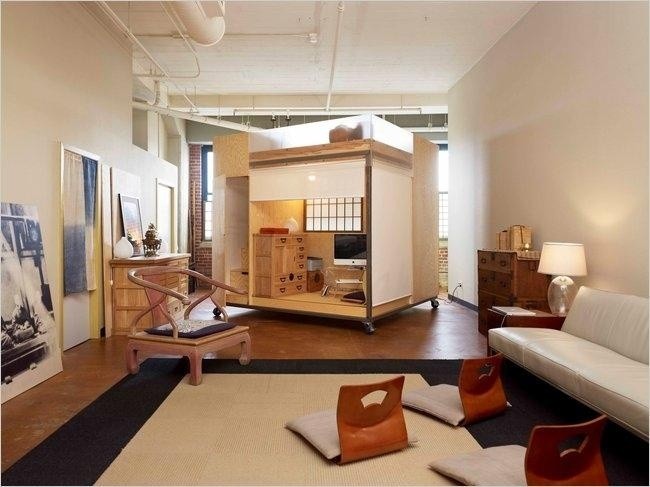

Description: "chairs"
405 354 507 425
427 415 610 487
283 376 408 466
125 268 251 385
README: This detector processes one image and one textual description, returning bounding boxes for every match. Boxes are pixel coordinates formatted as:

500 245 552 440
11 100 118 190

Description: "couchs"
488 287 650 447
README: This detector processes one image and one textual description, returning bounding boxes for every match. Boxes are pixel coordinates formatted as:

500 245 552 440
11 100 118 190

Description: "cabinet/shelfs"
109 253 191 335
477 247 552 339
252 233 309 294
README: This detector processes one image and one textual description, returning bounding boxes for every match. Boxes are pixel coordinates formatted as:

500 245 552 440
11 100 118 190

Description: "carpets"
92 373 488 487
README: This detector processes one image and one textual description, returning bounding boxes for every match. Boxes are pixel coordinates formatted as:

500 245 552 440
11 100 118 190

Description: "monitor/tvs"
333 232 368 267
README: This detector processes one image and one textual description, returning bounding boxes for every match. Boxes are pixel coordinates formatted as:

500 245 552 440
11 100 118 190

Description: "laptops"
492 306 536 317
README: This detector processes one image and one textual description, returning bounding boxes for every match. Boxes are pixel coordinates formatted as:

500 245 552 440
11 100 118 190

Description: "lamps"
537 242 588 317
